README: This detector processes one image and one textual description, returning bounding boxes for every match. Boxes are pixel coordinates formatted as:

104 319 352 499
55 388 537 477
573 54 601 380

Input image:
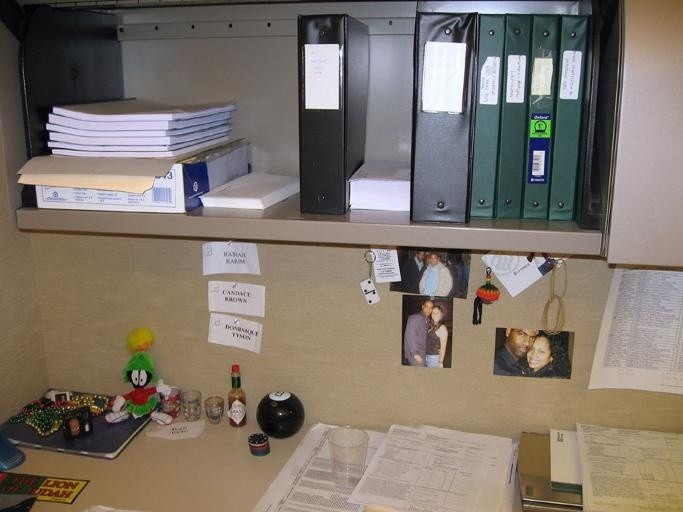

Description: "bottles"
159 387 180 420
227 366 248 426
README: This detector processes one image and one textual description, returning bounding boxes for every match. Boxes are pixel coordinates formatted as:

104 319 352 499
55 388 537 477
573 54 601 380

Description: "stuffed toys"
104 328 173 426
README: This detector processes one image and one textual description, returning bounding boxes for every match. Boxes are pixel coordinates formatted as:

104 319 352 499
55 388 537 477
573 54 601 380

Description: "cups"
326 425 368 486
181 390 201 422
205 396 225 426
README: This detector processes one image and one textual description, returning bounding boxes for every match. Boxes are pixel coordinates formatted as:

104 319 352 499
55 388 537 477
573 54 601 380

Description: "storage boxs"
16 140 249 214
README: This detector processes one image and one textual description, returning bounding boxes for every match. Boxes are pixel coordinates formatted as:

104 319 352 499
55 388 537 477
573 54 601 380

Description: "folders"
407 12 617 224
296 12 351 217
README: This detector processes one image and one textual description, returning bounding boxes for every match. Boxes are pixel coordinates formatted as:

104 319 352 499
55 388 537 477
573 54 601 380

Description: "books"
514 427 582 512
45 96 239 160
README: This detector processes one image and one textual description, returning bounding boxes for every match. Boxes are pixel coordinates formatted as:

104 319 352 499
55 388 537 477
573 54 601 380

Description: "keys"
364 250 376 279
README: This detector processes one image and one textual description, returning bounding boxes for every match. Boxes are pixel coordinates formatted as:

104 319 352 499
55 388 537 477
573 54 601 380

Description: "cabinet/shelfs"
1 0 682 268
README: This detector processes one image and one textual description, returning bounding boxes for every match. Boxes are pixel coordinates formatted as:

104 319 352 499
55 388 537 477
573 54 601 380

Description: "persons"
401 249 571 378
